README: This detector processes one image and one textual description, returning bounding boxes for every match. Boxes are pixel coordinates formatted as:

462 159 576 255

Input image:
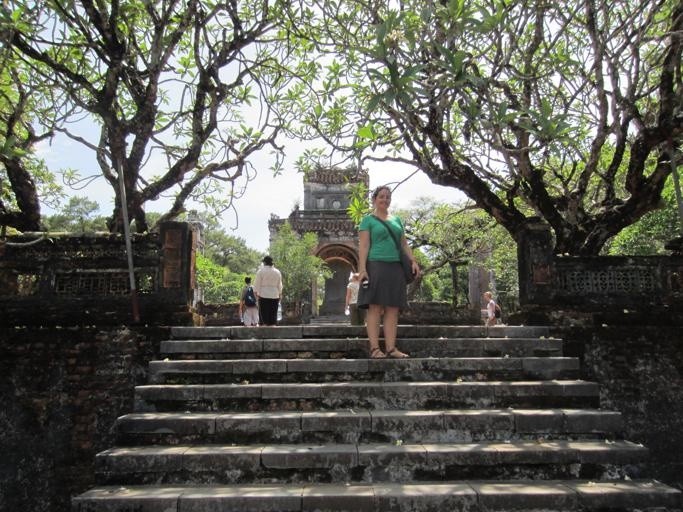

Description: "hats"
245 276 251 281
261 256 273 262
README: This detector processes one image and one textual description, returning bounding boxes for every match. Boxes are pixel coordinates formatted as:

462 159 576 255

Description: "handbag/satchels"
400 252 416 283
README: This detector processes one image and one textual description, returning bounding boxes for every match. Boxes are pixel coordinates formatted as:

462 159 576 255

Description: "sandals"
369 348 410 359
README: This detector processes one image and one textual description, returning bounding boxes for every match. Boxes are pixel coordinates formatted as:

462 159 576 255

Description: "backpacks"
495 304 501 318
245 286 257 307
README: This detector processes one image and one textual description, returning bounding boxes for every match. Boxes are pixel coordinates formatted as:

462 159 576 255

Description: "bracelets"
357 270 366 277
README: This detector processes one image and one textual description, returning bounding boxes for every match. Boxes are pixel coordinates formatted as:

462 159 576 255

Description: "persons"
240 277 260 326
483 291 497 326
357 185 422 359
346 273 365 325
253 255 283 326
